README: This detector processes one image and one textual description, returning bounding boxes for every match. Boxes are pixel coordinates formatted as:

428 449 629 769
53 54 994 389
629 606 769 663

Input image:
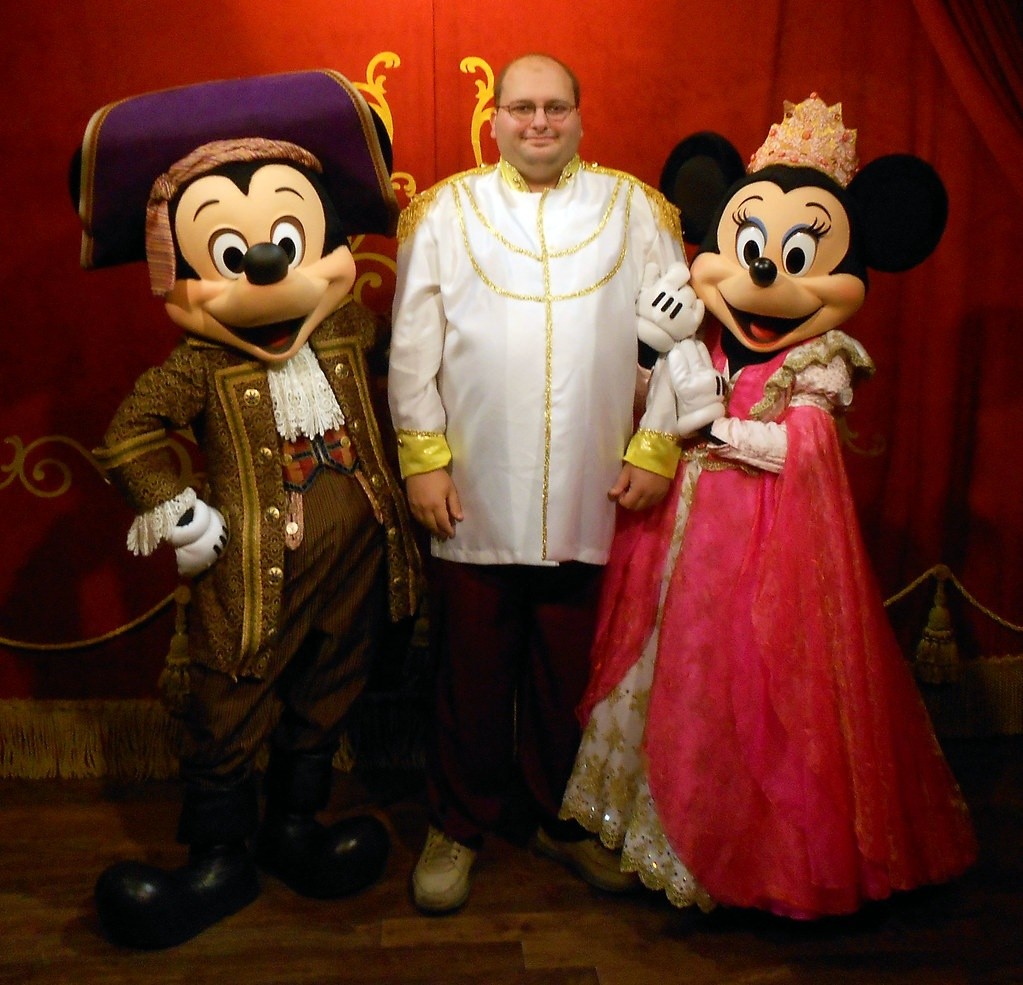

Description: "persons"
386 53 694 914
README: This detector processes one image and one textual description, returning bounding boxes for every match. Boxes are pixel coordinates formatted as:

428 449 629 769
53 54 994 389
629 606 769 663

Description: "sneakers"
412 824 478 913
535 826 640 893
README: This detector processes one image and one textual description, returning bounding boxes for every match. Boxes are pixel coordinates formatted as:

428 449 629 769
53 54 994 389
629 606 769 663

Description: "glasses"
497 99 575 121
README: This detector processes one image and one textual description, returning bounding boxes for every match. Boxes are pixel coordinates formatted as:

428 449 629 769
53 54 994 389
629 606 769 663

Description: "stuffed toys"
66 68 400 947
555 91 978 922
